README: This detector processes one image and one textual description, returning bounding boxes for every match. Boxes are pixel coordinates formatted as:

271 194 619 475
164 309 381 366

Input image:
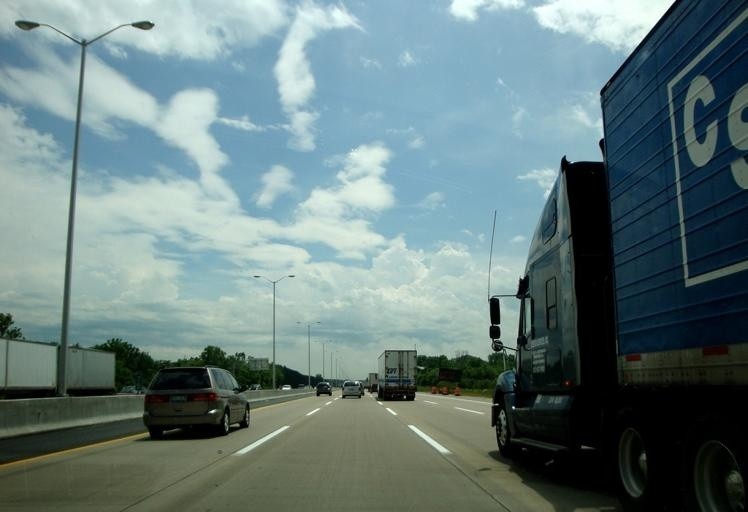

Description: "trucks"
486 0 744 512
364 350 417 401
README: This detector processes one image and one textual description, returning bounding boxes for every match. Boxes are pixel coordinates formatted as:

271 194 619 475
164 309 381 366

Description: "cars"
143 364 251 438
342 381 364 398
282 384 305 391
251 384 261 390
316 382 332 396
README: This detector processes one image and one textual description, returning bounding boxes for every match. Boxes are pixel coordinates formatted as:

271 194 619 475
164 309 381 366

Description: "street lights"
252 274 296 389
296 321 343 389
14 20 155 397
